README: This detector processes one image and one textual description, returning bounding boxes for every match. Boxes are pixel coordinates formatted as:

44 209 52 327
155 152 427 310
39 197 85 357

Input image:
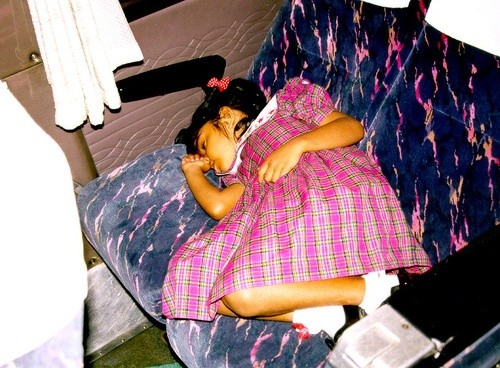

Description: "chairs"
73 0 498 368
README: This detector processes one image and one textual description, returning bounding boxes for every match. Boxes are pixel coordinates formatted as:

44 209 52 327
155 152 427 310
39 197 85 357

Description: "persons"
162 76 433 341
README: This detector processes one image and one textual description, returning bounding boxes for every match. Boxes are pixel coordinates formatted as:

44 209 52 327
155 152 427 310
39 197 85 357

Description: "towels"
26 0 144 131
